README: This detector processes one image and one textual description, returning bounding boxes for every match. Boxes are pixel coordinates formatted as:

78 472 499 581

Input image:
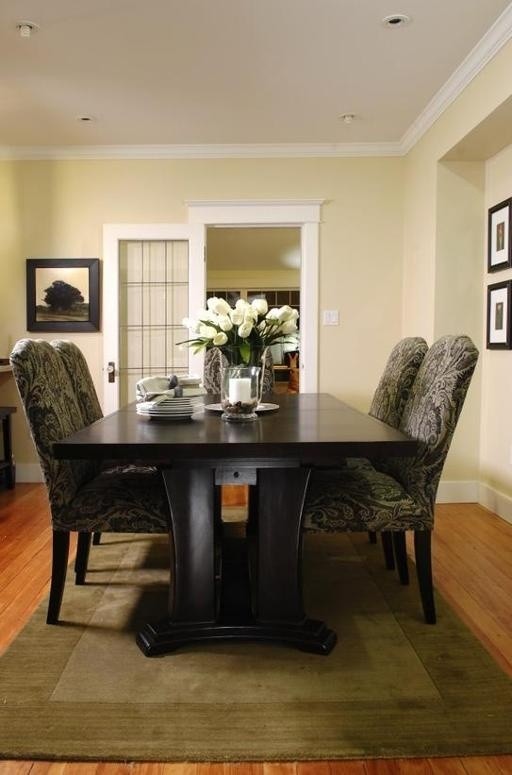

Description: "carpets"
0 505 511 761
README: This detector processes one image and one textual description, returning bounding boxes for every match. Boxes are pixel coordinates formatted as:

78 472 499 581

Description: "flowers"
173 296 302 366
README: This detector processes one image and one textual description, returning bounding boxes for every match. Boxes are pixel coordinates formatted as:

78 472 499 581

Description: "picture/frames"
487 196 512 351
25 258 101 332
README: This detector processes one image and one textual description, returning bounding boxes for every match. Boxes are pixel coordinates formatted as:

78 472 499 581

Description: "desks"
51 394 416 657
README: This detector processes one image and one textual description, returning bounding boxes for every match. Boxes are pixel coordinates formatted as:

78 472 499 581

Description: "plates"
136 395 205 423
205 402 282 412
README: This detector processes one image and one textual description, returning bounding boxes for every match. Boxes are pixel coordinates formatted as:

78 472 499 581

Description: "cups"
219 366 261 424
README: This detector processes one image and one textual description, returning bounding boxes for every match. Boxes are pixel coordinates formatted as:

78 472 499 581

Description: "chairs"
9 334 171 623
299 334 480 623
